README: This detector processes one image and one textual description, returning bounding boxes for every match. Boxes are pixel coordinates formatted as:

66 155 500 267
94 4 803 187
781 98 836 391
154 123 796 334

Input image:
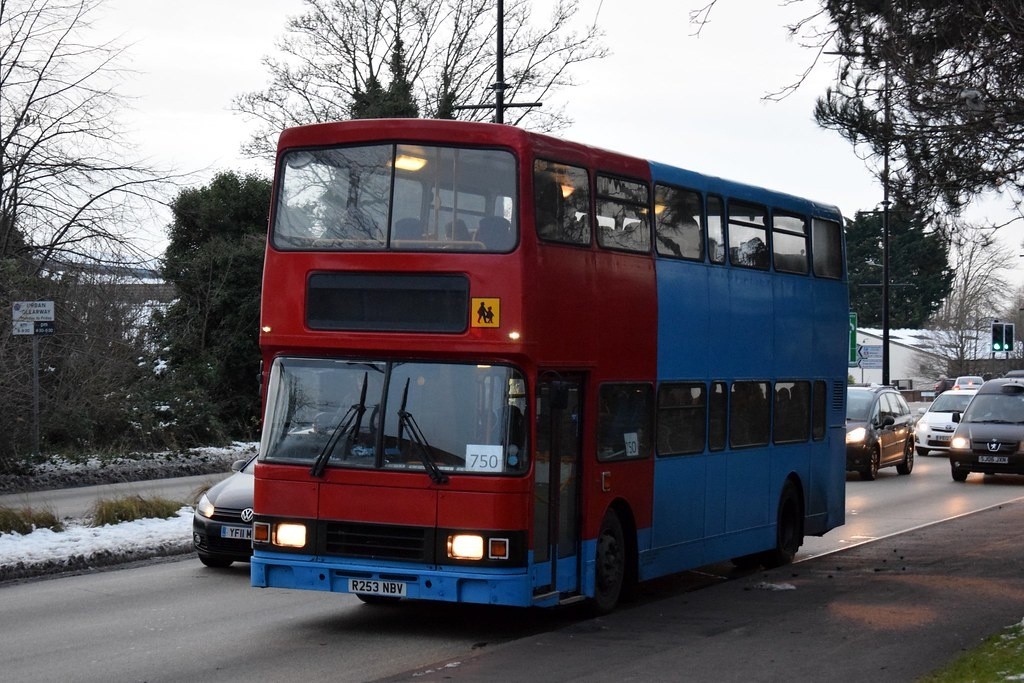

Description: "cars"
915 370 1024 480
193 453 259 567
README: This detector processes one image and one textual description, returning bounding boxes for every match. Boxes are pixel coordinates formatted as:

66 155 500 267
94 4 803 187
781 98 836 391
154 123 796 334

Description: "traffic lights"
992 324 1014 353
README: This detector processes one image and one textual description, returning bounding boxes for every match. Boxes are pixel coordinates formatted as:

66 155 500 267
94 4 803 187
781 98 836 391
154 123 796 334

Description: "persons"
562 185 774 267
328 363 836 483
934 374 949 397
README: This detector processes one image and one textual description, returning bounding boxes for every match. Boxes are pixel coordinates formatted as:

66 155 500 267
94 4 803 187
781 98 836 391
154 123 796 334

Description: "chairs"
396 217 514 243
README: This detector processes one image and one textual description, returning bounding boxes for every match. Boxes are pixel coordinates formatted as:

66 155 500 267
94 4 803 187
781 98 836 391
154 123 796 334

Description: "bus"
250 118 850 612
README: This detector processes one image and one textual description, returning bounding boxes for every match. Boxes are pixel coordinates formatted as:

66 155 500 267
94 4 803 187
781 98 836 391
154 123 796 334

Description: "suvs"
847 385 915 480
